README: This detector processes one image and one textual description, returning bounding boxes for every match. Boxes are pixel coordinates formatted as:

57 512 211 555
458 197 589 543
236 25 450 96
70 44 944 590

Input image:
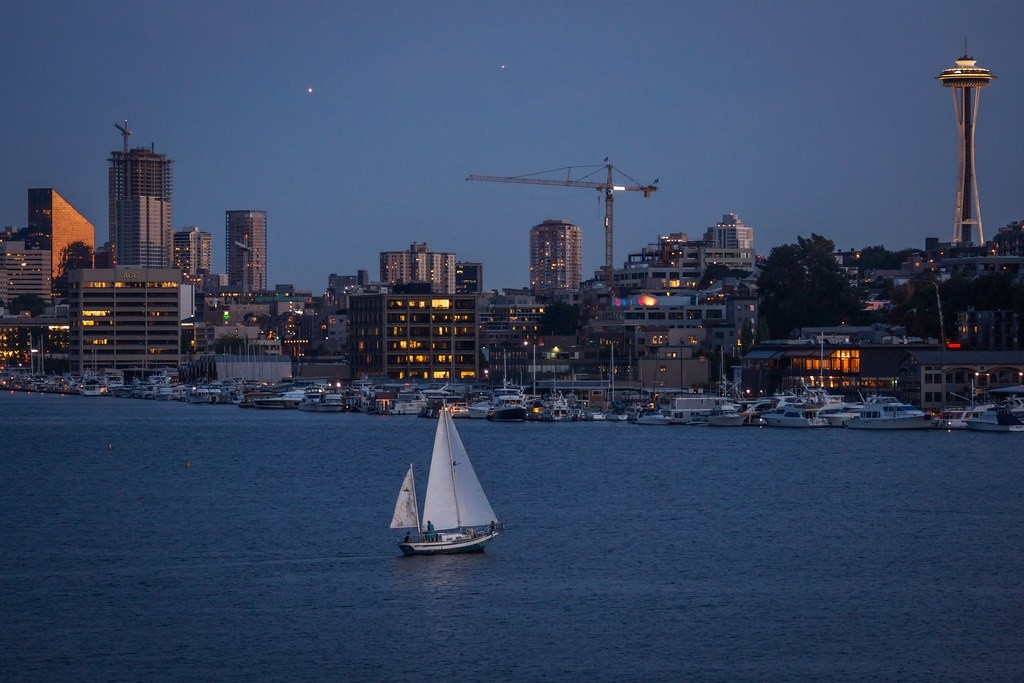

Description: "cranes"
462 153 662 294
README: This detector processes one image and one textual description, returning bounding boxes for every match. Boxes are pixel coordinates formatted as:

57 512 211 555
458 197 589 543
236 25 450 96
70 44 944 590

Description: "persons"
404 531 410 543
427 521 434 543
491 521 496 542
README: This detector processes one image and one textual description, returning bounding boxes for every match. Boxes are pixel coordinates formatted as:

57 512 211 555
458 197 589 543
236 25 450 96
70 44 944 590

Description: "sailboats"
389 398 509 555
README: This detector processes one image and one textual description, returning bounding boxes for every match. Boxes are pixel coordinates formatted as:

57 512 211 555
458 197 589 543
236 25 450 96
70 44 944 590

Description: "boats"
0 364 1024 436
